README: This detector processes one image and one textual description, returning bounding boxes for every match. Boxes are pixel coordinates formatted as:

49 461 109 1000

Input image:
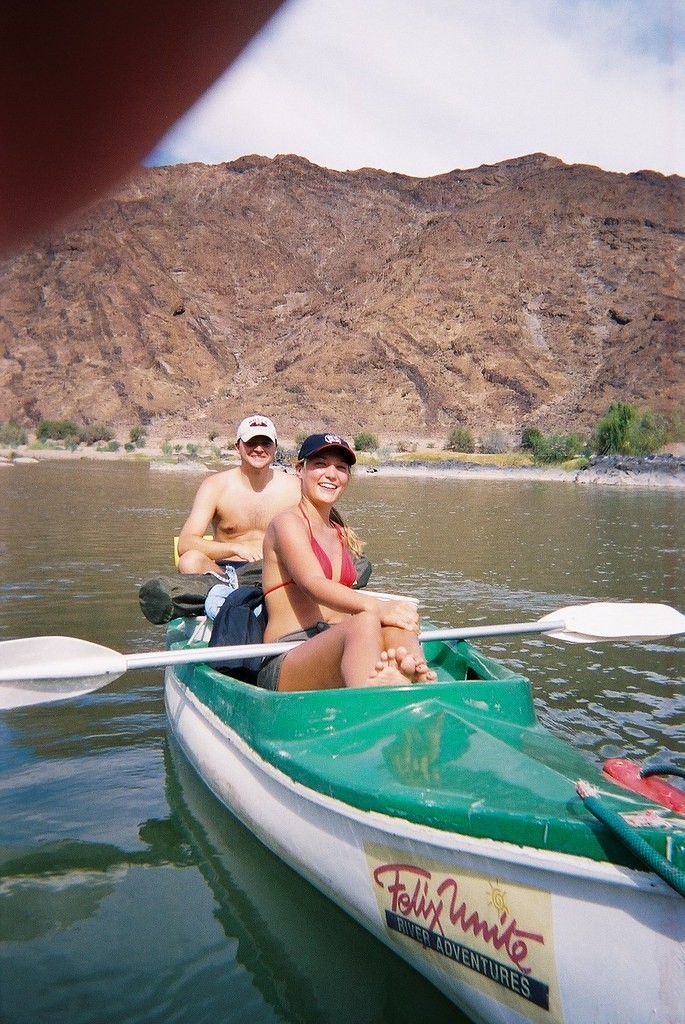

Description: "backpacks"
209 587 268 672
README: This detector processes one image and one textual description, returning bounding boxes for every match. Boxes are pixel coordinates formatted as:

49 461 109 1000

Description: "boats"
161 592 685 1024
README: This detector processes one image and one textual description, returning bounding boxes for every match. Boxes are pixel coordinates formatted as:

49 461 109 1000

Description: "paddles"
1 604 685 714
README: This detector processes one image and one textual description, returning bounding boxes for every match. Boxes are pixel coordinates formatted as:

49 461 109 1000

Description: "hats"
236 414 278 444
298 433 356 466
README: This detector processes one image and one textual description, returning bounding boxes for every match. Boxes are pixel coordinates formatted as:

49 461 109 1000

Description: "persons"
258 434 437 693
176 414 302 575
